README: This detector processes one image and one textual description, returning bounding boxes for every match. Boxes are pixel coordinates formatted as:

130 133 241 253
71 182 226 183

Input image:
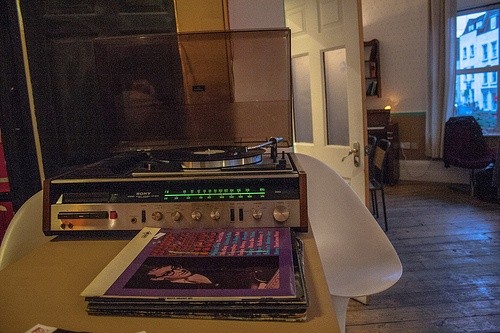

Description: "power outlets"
399 142 418 150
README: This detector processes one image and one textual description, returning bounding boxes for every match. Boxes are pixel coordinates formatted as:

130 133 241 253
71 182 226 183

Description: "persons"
143 262 267 289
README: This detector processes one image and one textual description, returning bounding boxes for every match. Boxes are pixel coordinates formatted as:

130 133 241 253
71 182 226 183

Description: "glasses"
155 265 182 277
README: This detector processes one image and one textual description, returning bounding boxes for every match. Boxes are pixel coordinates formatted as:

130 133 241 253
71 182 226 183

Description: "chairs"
443 116 495 197
367 135 392 232
